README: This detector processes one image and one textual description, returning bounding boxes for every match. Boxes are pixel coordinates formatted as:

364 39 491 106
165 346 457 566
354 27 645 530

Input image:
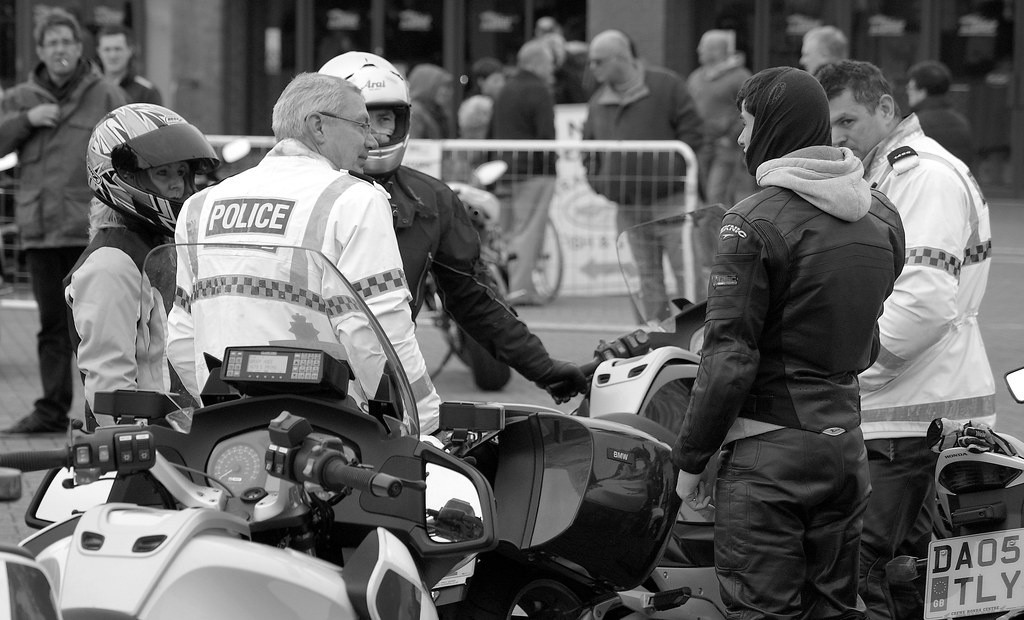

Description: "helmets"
86 103 220 238
318 51 413 176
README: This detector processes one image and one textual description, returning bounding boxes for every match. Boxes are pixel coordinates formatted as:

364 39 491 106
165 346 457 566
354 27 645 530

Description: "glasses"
305 111 372 137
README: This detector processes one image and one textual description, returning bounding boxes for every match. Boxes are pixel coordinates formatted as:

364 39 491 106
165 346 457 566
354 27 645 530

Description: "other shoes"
0 411 68 439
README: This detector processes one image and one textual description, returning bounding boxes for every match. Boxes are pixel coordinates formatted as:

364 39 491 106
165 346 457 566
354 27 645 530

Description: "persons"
811 60 996 620
317 50 588 405
61 102 220 434
0 7 163 437
671 66 905 620
684 29 752 296
800 26 849 73
407 62 451 142
906 59 976 177
458 16 708 322
167 71 449 447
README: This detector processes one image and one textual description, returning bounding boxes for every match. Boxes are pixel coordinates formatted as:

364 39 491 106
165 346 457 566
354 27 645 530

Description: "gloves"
536 359 588 405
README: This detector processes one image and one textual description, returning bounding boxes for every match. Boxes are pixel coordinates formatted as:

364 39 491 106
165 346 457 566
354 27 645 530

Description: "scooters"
1 152 1023 620
